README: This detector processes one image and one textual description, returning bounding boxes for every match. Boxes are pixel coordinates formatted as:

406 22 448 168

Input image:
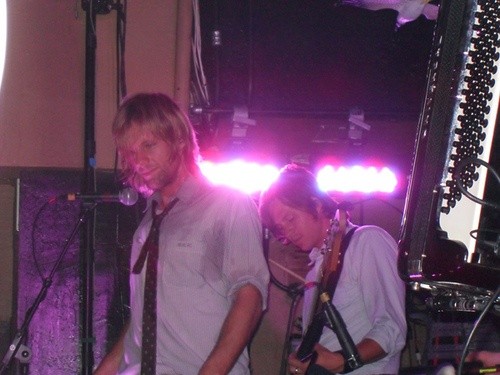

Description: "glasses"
119 137 163 162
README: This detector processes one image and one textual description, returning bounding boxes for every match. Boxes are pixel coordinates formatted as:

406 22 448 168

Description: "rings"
295 367 300 373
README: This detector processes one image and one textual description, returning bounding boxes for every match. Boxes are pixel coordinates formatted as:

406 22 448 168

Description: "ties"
131 197 181 374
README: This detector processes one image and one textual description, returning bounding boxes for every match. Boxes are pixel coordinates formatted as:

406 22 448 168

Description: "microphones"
316 283 364 369
56 189 138 206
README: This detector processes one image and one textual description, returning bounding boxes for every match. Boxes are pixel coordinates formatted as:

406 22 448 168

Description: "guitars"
290 206 346 375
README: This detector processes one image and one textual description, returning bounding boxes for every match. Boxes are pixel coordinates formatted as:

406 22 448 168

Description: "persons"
93 92 273 375
399 239 470 370
257 163 409 375
463 349 500 368
